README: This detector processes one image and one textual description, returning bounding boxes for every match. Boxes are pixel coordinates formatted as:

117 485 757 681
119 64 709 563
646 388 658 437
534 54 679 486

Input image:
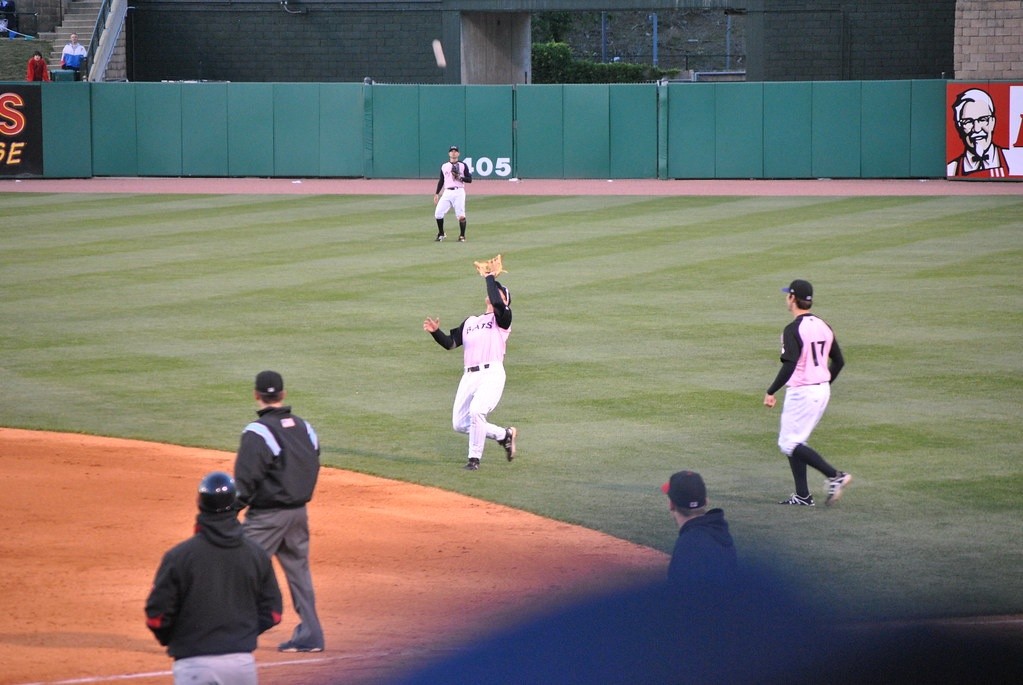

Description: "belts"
448 187 458 191
467 364 487 371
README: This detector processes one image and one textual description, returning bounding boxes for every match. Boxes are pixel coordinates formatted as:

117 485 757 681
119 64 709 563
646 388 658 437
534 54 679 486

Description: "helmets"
197 473 235 513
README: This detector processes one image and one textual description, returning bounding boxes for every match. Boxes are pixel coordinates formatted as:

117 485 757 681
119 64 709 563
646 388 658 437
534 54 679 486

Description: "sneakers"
459 235 466 241
778 490 815 506
434 232 446 241
825 472 852 504
462 458 478 471
277 637 323 654
498 427 516 461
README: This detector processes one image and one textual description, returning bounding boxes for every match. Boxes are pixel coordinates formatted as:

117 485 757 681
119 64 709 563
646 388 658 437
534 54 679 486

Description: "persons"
660 470 736 604
143 471 283 685
423 254 516 470
60 33 87 81
25 50 53 82
234 371 327 652
433 144 472 242
764 280 852 506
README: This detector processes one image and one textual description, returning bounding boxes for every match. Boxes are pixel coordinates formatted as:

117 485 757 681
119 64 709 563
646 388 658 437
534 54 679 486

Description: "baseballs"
432 38 447 67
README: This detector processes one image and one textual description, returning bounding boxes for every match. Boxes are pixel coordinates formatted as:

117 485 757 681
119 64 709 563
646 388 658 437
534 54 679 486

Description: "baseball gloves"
451 162 461 179
474 255 503 279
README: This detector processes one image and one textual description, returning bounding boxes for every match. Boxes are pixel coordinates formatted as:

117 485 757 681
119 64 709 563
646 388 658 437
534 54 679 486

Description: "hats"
448 145 459 153
256 370 283 395
782 279 812 302
496 280 511 307
659 468 705 508
